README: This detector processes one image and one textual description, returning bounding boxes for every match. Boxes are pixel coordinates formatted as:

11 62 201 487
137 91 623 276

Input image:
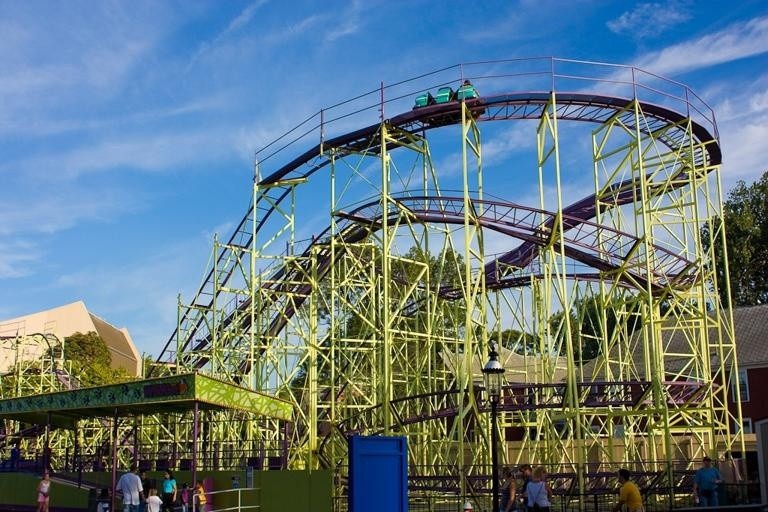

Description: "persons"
693 456 724 508
116 464 207 512
463 80 471 86
34 473 52 512
612 468 645 512
499 464 554 512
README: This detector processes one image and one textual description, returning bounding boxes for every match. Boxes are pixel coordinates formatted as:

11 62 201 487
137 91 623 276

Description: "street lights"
481 344 505 511
